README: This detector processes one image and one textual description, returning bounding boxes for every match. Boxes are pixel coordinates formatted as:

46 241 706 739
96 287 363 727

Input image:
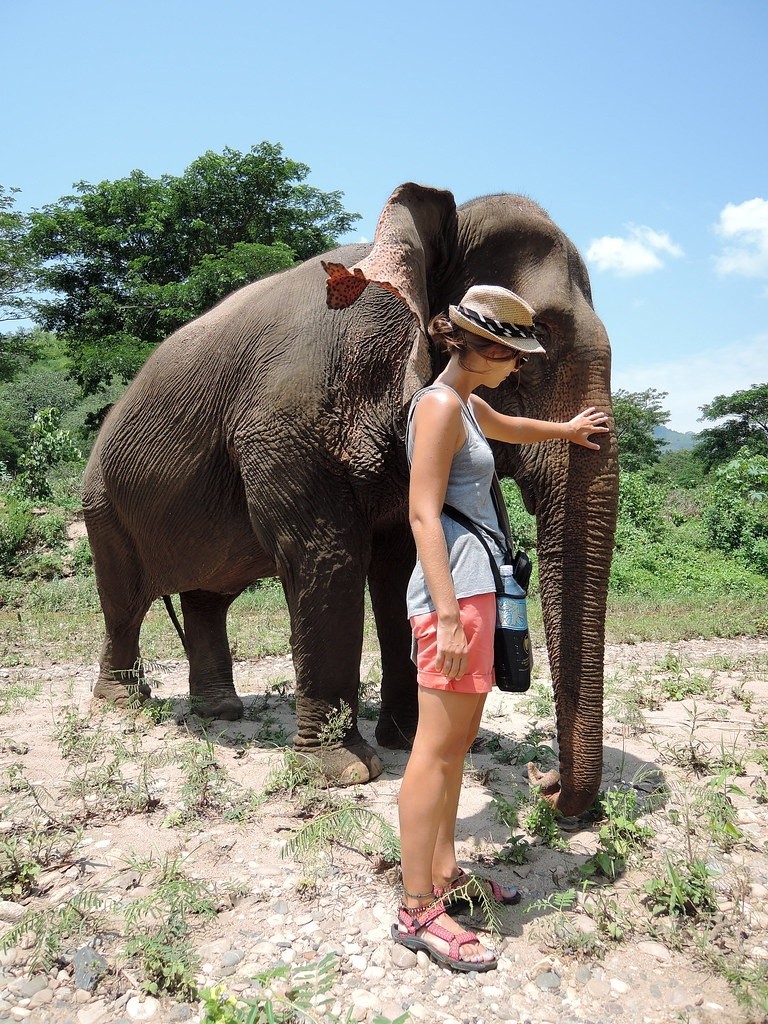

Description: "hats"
450 284 547 354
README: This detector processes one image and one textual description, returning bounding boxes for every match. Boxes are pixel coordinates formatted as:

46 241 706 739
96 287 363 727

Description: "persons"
389 283 611 972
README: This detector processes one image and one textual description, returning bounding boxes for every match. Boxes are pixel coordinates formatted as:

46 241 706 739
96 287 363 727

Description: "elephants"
79 184 618 827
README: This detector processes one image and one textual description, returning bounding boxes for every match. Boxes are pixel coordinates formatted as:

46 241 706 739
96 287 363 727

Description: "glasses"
507 350 530 371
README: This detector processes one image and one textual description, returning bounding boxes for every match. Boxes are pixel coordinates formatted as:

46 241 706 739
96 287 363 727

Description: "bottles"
497 564 527 597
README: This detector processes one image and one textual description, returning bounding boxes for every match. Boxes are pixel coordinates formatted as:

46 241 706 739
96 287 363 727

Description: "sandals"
431 866 521 920
391 899 498 973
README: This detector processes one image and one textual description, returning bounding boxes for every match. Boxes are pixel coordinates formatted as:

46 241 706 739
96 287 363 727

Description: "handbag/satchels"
495 550 533 693
